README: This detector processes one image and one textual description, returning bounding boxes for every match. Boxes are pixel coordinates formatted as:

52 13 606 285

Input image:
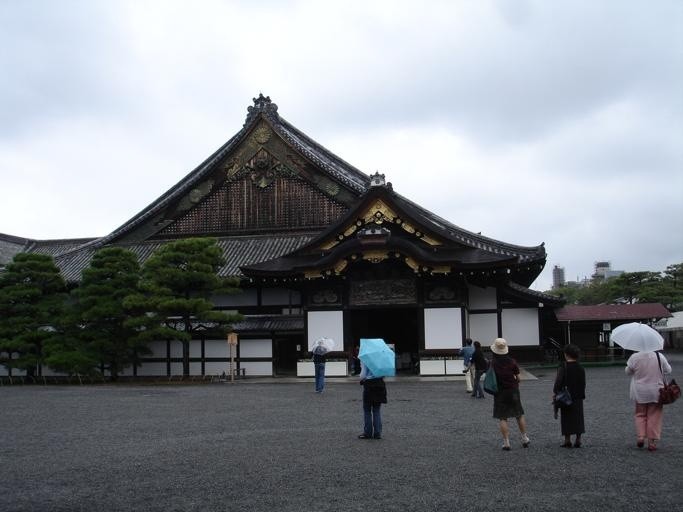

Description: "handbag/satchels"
657 379 682 404
483 359 499 396
555 387 572 406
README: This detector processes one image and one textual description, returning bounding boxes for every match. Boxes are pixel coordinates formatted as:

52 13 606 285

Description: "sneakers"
358 433 383 439
502 443 511 451
520 435 531 447
560 441 582 448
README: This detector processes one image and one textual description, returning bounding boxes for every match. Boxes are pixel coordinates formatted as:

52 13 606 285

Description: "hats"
490 337 509 356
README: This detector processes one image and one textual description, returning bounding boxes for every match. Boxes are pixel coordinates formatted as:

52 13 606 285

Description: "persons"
483 337 530 449
358 364 387 441
458 337 476 393
624 350 672 451
462 341 487 399
313 338 327 395
553 344 586 448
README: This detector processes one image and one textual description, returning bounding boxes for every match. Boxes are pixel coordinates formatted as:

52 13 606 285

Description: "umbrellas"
357 335 396 380
609 321 665 353
